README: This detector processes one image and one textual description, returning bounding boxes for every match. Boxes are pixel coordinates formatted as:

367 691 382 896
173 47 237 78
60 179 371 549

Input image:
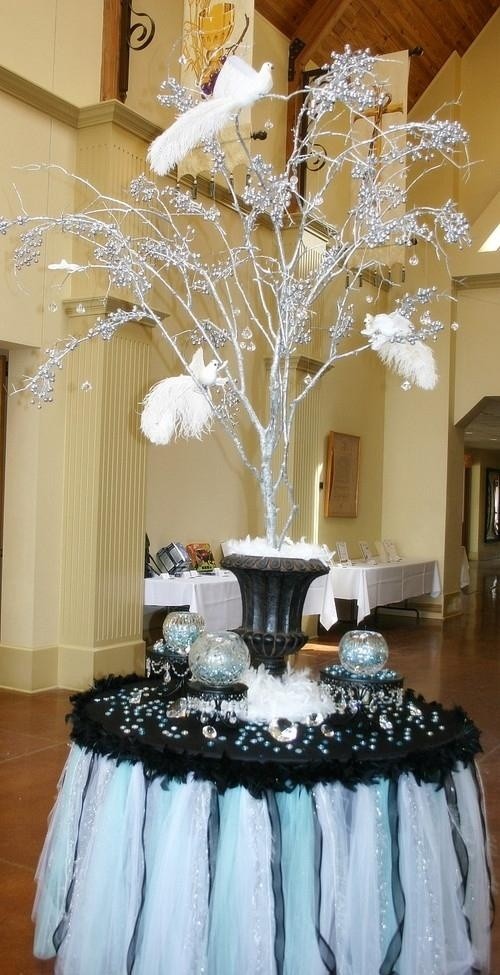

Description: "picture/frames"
324 430 361 519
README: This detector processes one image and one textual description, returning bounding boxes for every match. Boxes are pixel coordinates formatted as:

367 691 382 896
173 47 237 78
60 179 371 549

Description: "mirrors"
485 467 500 543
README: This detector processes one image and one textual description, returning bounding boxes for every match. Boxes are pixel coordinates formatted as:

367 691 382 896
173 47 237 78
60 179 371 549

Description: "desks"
145 571 339 632
330 556 442 627
31 673 499 975
